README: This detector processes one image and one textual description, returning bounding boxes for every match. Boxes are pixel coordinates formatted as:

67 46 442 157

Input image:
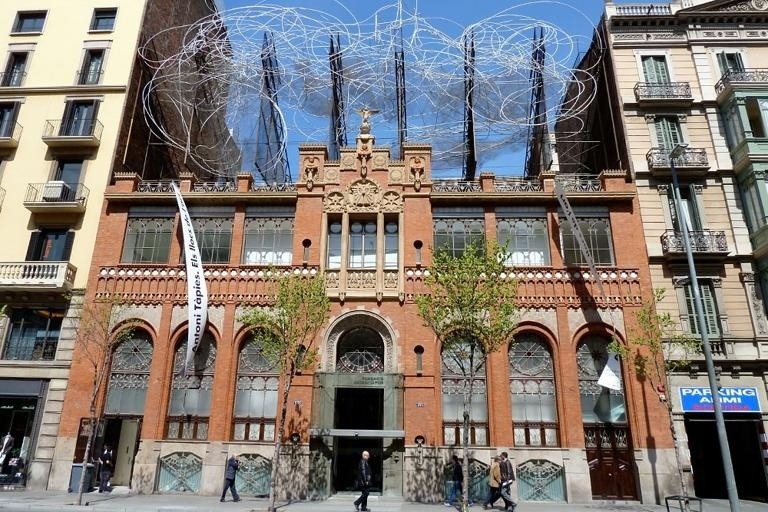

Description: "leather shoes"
354 502 371 512
220 498 241 502
484 503 493 508
505 501 517 512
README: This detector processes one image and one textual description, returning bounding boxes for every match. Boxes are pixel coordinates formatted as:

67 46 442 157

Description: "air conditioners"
40 180 71 200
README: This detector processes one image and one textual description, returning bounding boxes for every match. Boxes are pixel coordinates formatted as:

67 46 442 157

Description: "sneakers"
444 502 453 507
468 503 473 506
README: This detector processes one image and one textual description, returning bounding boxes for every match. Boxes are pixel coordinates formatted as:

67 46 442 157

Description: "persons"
444 455 474 507
220 453 242 502
98 445 114 493
483 452 517 512
354 451 371 512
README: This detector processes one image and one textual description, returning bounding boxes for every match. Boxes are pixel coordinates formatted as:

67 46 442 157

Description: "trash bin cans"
68 463 94 493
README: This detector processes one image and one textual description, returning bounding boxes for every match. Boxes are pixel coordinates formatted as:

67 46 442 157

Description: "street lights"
669 143 740 512
